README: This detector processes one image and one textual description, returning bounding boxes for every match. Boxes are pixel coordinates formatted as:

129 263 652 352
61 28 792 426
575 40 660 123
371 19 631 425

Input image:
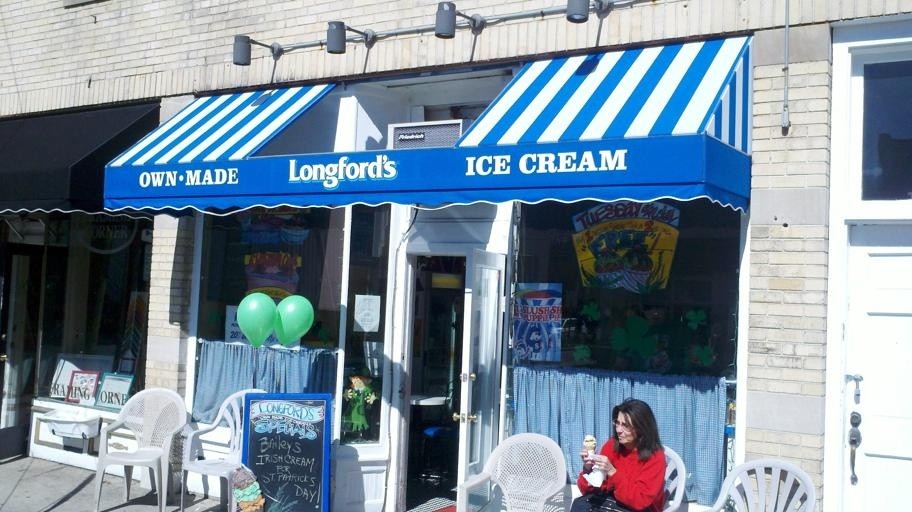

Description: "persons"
571 395 668 512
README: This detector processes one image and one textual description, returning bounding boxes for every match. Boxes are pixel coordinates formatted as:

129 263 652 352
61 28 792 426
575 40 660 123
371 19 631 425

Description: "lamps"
232 0 615 65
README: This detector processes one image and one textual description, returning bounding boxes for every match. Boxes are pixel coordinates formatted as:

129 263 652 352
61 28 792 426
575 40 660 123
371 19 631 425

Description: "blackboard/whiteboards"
241 393 330 512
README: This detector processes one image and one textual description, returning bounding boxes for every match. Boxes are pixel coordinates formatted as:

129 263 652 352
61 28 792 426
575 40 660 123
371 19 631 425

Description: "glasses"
613 420 633 431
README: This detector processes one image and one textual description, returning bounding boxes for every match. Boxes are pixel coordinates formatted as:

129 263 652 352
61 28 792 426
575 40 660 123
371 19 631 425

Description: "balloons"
275 294 316 347
236 291 278 352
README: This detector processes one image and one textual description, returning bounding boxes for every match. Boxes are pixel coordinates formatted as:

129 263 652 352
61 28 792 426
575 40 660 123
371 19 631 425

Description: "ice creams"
231 465 266 512
583 435 597 450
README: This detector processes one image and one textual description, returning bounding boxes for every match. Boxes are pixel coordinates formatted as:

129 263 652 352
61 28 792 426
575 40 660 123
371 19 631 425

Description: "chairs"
93 386 266 512
659 445 685 512
703 459 815 512
458 432 567 512
